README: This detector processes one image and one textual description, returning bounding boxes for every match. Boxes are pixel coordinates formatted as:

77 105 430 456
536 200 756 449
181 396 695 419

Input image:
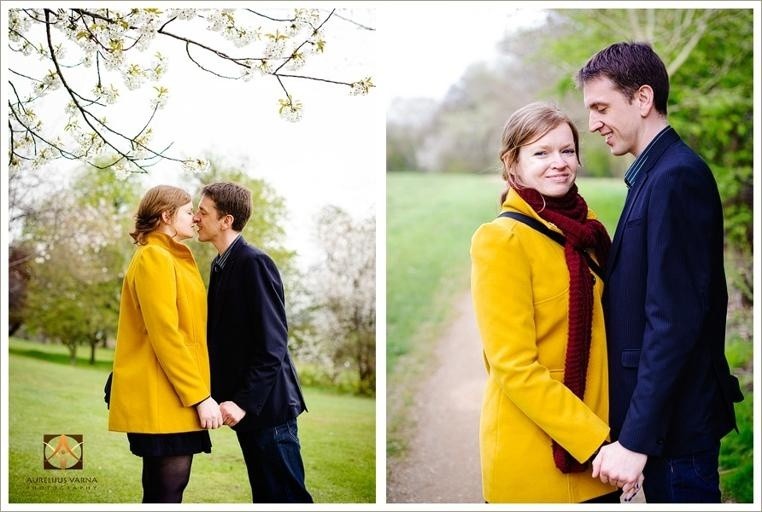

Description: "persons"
467 99 644 504
190 183 316 504
573 40 746 501
106 185 226 504
